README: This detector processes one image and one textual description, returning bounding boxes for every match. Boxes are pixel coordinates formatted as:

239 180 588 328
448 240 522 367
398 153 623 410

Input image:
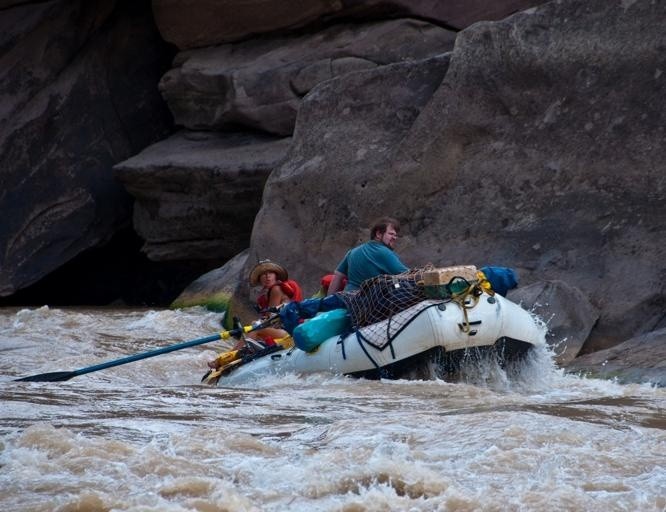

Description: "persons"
326 217 412 293
206 258 305 370
310 276 346 300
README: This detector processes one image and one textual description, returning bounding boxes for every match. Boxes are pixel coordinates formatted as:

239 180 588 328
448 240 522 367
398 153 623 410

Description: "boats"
215 276 546 392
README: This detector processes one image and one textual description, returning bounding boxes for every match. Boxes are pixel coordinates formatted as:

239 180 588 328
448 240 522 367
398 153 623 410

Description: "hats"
249 259 289 288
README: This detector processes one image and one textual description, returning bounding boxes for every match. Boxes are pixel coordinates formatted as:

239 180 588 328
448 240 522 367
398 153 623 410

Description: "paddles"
15 321 266 381
